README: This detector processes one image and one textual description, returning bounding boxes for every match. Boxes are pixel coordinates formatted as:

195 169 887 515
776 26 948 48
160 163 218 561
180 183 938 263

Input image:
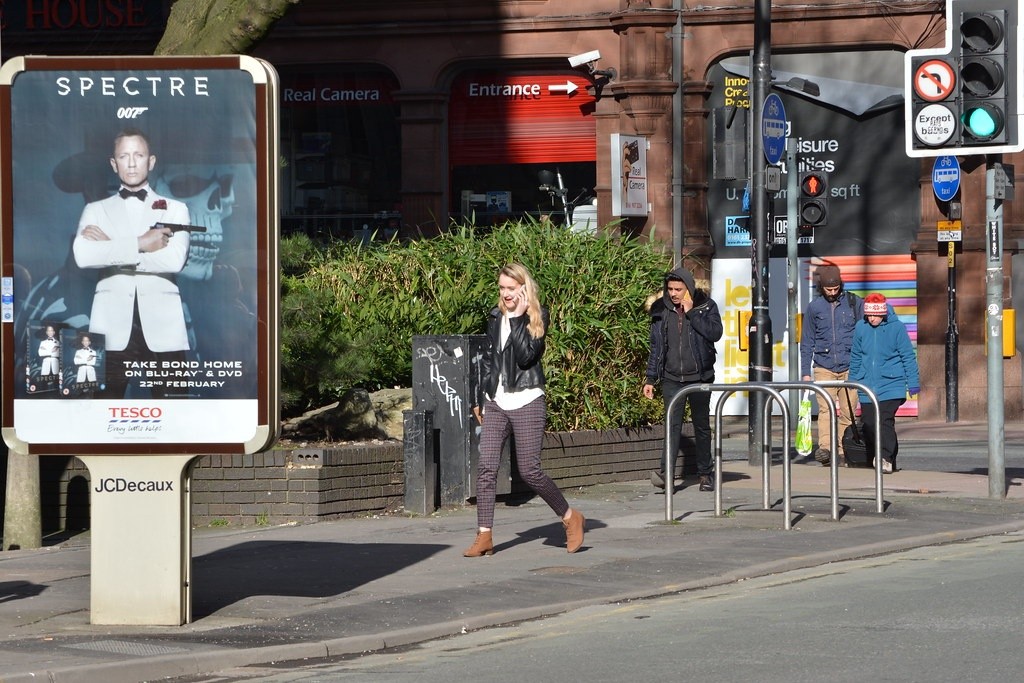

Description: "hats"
820 271 841 287
864 293 888 315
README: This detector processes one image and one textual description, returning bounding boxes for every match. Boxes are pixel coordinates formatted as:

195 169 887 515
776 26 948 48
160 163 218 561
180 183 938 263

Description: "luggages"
842 387 875 469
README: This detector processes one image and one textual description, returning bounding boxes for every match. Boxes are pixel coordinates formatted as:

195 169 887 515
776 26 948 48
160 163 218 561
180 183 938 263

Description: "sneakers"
463 531 493 557
562 508 586 553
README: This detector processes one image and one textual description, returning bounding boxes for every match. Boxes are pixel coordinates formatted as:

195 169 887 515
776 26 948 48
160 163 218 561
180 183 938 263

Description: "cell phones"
684 290 690 300
513 284 527 306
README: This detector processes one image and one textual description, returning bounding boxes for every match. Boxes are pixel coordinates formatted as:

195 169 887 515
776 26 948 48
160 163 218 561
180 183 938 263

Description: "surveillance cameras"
566 49 601 68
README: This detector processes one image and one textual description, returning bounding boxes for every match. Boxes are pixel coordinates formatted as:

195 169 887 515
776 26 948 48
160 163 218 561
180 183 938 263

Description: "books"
58 327 106 397
25 320 69 393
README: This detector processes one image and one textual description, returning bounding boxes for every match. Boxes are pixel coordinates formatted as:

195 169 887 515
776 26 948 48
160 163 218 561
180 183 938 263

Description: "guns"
150 222 207 237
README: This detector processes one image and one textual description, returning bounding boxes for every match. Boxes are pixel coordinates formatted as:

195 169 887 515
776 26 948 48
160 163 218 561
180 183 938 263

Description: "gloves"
909 388 921 398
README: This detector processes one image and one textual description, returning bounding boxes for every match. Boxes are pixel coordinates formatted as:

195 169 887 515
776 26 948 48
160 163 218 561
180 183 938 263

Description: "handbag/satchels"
795 388 812 457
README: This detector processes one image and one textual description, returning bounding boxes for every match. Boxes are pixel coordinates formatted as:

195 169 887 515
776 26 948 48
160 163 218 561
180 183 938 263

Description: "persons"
848 293 921 473
74 335 100 398
38 325 60 391
72 129 191 400
464 263 587 557
644 267 724 491
800 266 865 468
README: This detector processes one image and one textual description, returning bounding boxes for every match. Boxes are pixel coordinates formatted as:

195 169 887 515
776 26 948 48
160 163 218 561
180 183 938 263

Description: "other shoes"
699 475 714 491
815 449 832 467
882 457 892 473
651 471 674 490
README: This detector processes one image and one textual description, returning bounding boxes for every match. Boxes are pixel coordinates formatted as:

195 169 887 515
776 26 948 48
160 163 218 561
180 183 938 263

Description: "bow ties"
48 339 53 341
118 188 148 201
83 347 89 350
